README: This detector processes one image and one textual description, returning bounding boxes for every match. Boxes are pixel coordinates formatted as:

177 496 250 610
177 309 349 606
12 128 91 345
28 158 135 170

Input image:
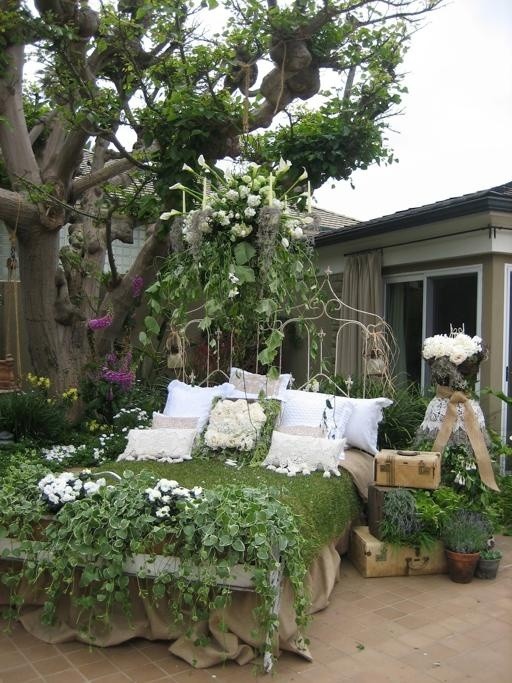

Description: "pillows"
121 367 391 480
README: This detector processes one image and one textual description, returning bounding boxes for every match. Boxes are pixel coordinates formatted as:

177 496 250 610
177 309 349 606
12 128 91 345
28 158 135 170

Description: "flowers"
418 330 486 365
129 151 343 366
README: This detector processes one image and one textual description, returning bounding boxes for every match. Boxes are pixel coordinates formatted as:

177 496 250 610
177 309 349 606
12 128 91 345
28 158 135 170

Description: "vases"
429 351 484 391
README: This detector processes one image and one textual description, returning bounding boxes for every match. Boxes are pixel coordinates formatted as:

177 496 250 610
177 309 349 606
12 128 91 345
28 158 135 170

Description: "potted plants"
438 505 494 582
473 550 504 580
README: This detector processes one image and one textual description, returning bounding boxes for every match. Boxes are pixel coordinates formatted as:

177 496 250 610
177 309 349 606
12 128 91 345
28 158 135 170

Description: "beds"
1 270 406 670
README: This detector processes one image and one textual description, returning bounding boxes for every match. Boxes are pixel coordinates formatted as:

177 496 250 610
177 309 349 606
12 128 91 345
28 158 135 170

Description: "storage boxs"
365 484 425 541
371 448 441 489
347 524 447 578
